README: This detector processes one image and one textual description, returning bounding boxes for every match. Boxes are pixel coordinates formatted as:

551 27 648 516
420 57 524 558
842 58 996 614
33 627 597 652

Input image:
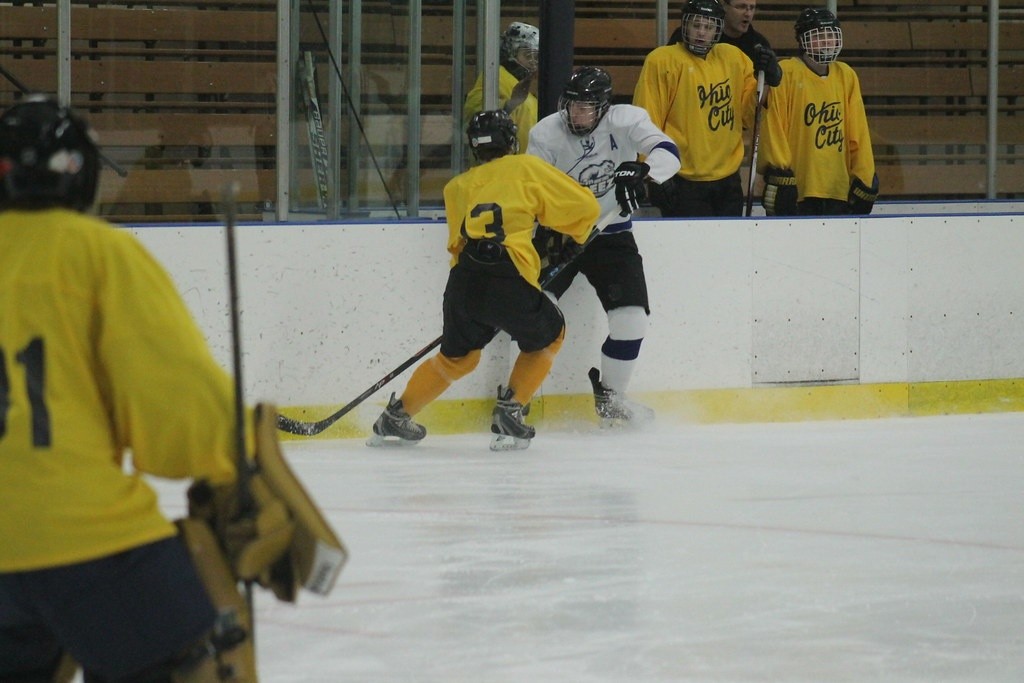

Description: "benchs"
0 0 1024 230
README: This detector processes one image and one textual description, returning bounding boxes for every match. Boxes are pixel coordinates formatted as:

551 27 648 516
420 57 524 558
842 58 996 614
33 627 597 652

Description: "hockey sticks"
745 68 766 217
223 203 256 632
271 334 447 436
297 49 343 210
541 202 624 290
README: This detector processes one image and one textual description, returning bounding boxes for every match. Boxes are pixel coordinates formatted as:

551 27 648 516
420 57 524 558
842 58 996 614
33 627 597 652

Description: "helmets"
682 0 725 55
466 109 517 162
0 94 99 212
562 66 612 136
795 9 840 64
501 22 539 59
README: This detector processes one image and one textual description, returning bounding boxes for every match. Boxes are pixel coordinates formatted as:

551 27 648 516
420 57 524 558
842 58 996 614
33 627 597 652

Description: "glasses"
725 0 756 12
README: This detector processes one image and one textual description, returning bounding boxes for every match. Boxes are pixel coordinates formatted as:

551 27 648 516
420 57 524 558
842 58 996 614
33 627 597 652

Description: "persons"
756 8 880 216
632 0 782 218
366 110 602 450
0 94 254 683
525 67 683 431
667 0 771 59
463 23 539 165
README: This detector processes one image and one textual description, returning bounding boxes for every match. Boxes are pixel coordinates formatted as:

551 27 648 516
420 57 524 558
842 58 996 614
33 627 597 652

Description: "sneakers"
366 391 427 447
490 385 535 450
589 367 657 430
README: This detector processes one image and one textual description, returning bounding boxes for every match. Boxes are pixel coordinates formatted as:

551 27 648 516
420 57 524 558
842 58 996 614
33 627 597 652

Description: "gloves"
761 167 798 216
187 472 297 601
614 161 651 213
847 173 879 215
753 48 782 87
650 180 683 212
561 237 585 263
